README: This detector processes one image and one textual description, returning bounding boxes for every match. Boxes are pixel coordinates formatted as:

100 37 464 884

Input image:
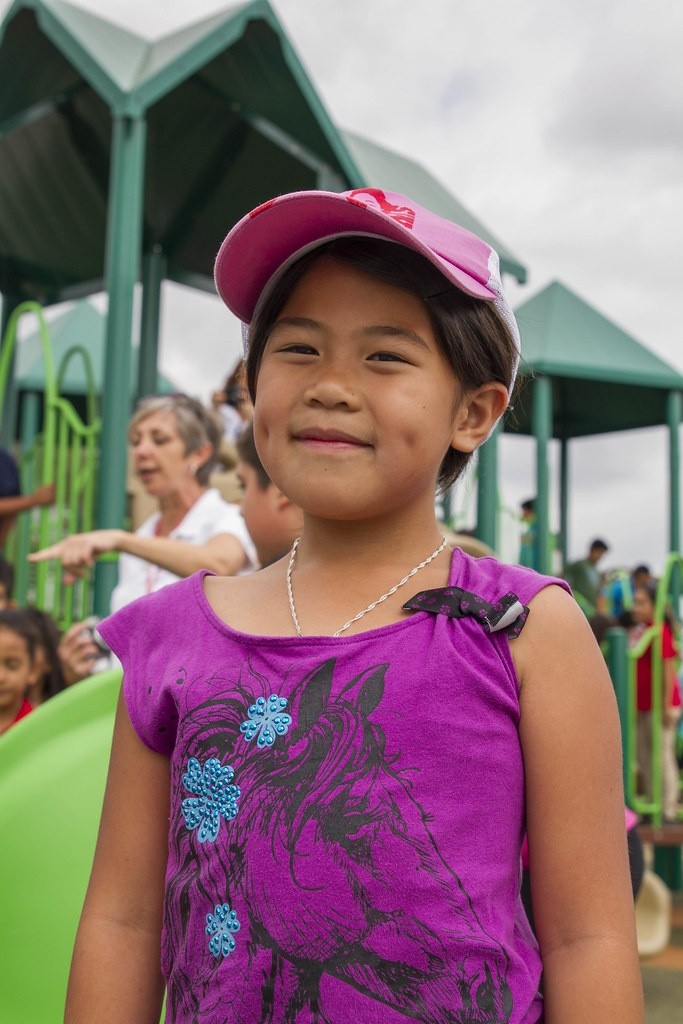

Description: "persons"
515 496 683 823
0 360 307 734
62 184 643 1024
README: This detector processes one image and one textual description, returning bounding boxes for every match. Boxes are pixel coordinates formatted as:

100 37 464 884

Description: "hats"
213 187 521 451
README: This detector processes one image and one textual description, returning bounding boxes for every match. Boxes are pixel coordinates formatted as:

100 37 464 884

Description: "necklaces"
285 534 449 637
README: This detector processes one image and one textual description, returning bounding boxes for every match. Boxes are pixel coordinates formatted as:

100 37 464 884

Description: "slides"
0 666 170 1024
